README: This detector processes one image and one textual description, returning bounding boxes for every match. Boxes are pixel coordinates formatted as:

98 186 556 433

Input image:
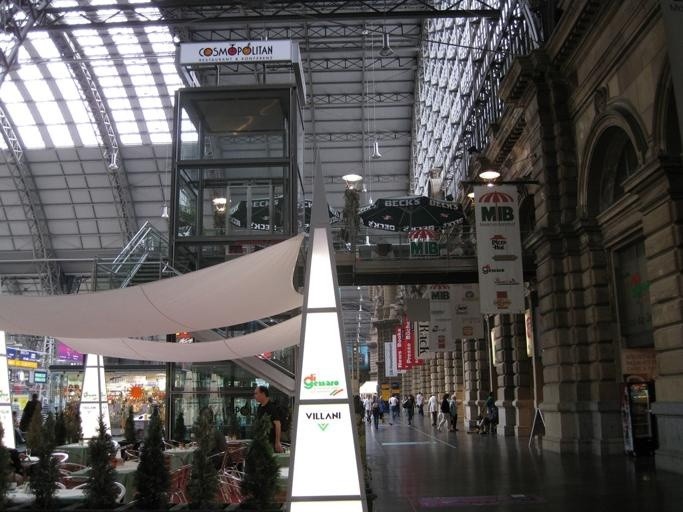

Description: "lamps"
368 0 384 160
107 146 119 170
158 152 169 220
379 0 393 59
352 283 362 347
359 115 374 246
473 154 501 183
341 170 364 190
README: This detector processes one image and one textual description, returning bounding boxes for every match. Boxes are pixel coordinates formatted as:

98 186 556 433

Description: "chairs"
0 412 256 510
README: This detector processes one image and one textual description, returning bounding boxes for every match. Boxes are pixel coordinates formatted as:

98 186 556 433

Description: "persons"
120 391 227 439
15 389 46 434
251 386 285 454
361 391 500 436
